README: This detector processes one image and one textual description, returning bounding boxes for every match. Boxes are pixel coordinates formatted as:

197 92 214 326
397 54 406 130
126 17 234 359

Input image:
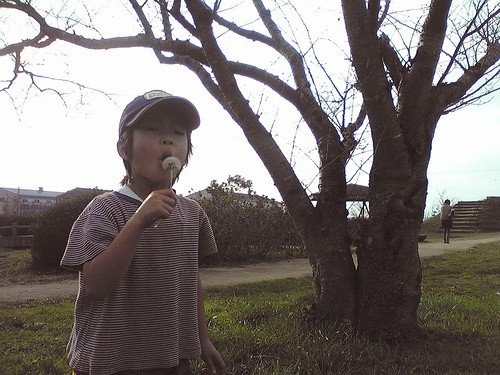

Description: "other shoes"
444 241 449 243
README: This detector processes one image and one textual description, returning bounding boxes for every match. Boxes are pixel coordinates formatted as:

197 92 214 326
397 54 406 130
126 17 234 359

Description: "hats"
118 90 201 134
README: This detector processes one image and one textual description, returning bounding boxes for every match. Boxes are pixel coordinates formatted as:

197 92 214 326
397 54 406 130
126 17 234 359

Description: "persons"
59 90 226 375
439 199 454 243
